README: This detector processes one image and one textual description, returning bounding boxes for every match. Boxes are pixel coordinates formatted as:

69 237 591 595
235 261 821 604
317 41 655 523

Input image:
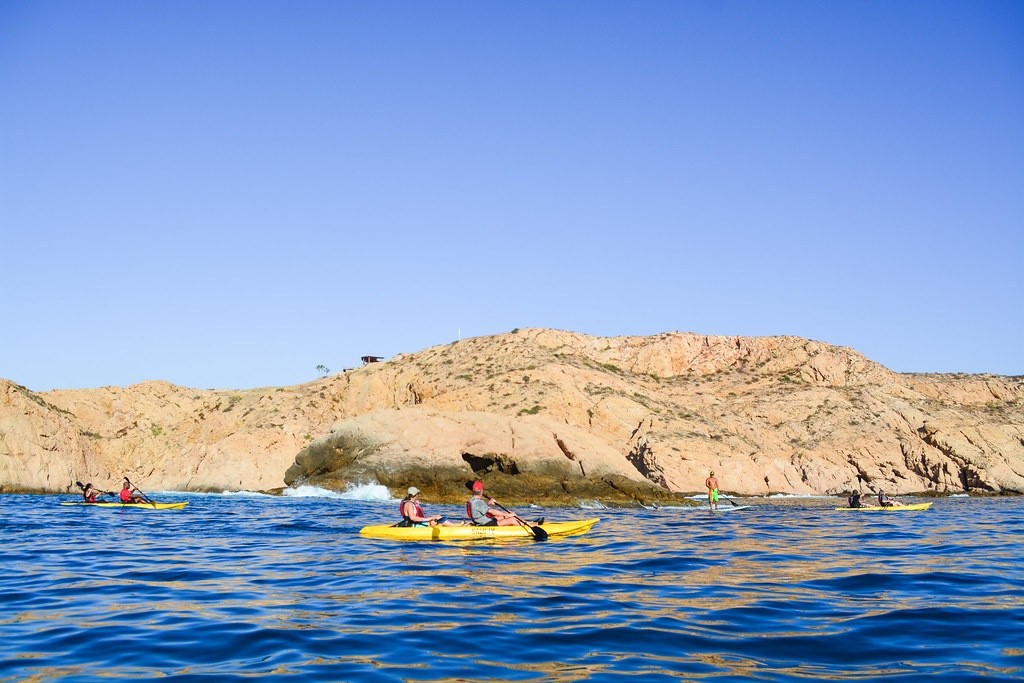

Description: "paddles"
712 488 739 507
436 516 448 525
76 480 116 498
124 476 156 509
465 479 548 540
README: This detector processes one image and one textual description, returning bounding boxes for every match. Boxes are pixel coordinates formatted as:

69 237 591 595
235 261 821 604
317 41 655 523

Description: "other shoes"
534 518 540 522
539 517 545 524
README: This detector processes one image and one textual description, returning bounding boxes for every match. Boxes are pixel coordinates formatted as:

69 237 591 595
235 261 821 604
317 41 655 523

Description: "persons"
706 470 720 510
399 487 466 527
467 481 545 526
878 489 908 506
119 482 149 504
848 489 878 507
83 483 109 503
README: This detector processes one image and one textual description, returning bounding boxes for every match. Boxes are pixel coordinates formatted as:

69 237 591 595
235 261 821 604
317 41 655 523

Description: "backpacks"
848 495 855 504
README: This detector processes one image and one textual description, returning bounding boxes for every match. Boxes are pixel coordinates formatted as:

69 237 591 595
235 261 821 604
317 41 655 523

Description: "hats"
407 487 421 496
710 471 715 475
473 481 483 490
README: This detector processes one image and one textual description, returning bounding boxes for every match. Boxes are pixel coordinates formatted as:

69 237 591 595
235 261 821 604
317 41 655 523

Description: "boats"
358 517 601 541
61 501 189 510
636 499 759 512
835 502 933 510
